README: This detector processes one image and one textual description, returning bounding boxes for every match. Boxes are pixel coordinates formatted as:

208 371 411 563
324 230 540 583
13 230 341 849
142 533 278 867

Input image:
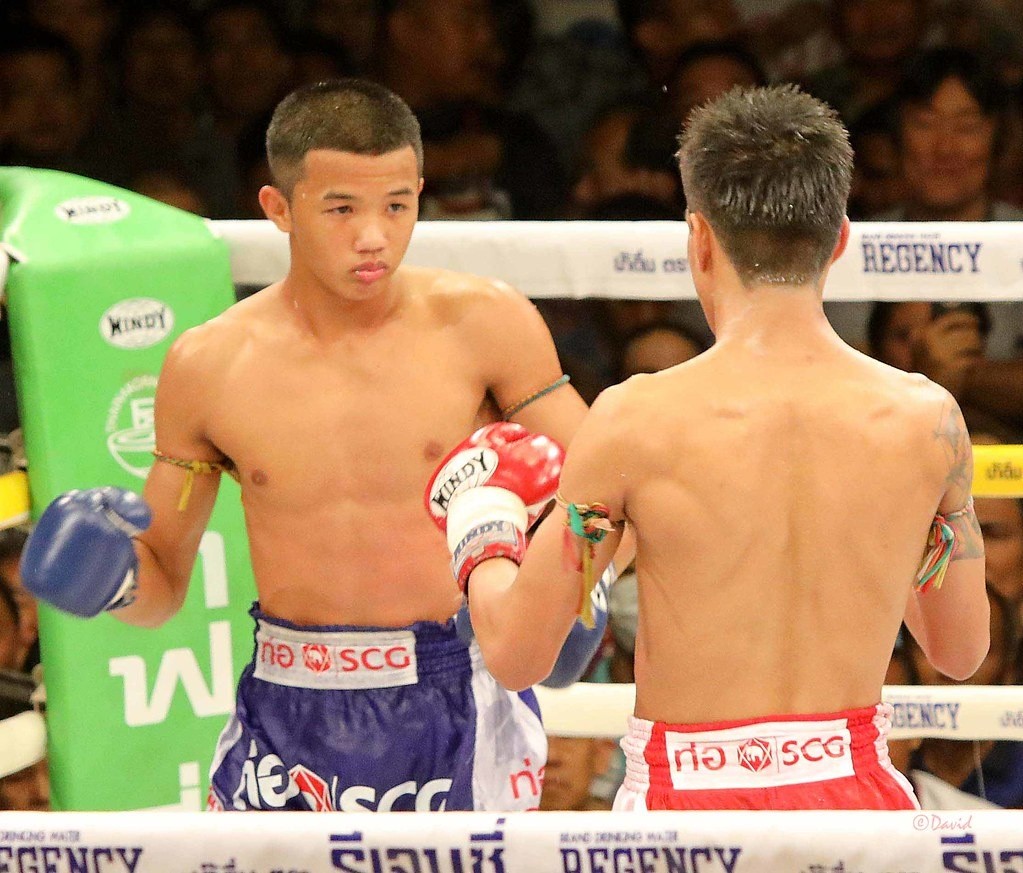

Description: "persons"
902 579 1023 810
0 758 49 811
971 495 1023 672
886 646 1006 810
2 2 1022 446
539 736 618 812
19 81 636 812
0 575 20 669
3 528 39 673
427 81 990 810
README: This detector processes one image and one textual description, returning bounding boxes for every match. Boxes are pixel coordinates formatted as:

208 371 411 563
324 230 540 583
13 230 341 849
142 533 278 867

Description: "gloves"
20 488 153 620
423 419 568 578
